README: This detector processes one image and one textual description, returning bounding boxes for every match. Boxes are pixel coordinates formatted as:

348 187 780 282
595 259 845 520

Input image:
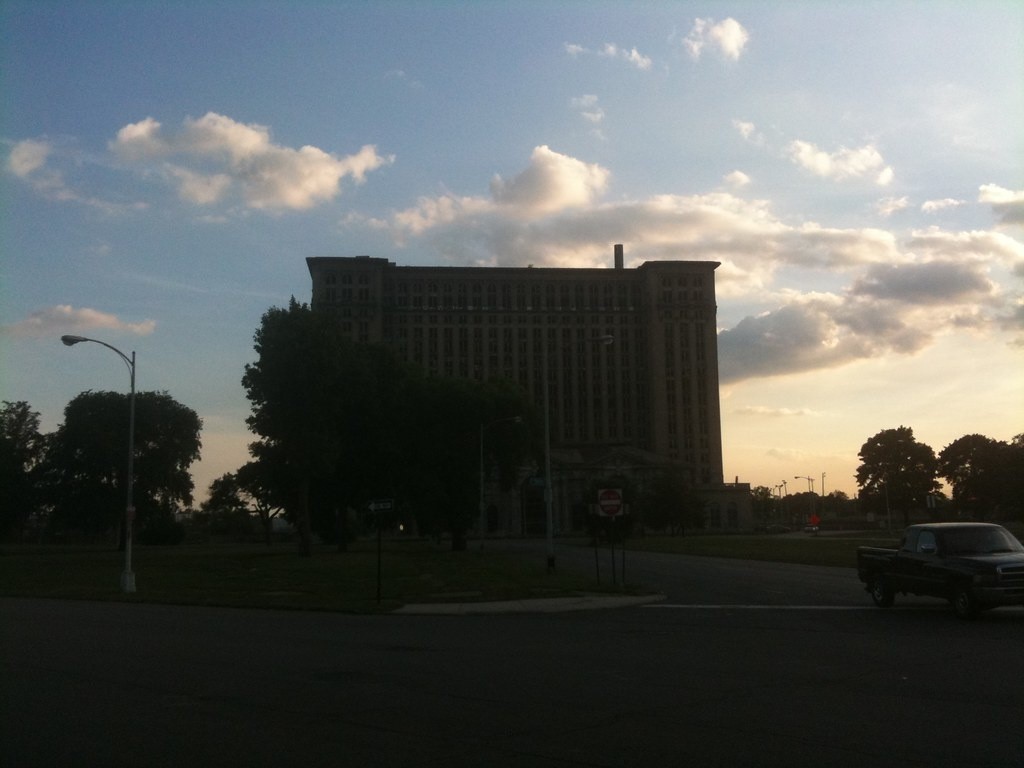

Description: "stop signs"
599 488 624 516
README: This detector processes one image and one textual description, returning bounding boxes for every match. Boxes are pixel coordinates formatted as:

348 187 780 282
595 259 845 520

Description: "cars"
771 525 791 533
805 524 819 532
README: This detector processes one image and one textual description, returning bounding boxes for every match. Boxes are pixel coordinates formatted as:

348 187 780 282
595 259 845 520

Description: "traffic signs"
367 500 393 511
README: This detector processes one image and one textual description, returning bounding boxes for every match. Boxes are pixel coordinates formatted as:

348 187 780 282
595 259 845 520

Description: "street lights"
61 334 145 606
479 415 524 550
794 476 817 523
540 334 615 574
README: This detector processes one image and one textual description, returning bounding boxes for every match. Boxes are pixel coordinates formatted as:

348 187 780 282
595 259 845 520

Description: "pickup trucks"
857 522 1024 619
